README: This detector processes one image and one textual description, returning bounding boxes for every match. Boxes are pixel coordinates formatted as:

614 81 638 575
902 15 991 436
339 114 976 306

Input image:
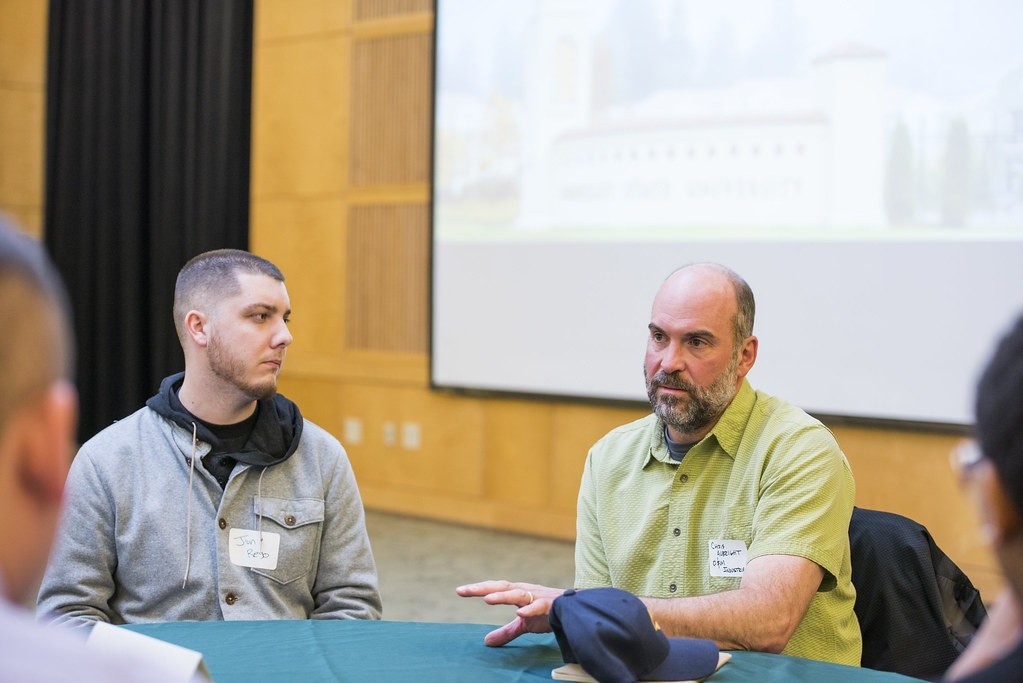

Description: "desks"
116 619 932 683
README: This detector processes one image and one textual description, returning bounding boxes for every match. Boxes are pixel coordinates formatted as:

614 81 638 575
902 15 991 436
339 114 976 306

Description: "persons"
456 263 862 667
36 250 383 628
0 227 207 683
939 311 1023 683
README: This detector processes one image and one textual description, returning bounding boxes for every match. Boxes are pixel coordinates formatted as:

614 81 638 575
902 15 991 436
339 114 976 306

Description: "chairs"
849 506 988 683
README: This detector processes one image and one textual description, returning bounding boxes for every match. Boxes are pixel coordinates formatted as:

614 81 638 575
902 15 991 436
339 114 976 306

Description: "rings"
528 592 533 603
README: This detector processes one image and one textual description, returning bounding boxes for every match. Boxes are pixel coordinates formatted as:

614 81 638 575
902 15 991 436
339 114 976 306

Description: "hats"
547 588 720 683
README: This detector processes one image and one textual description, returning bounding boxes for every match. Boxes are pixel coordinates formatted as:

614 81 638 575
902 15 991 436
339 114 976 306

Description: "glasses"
949 439 991 494
